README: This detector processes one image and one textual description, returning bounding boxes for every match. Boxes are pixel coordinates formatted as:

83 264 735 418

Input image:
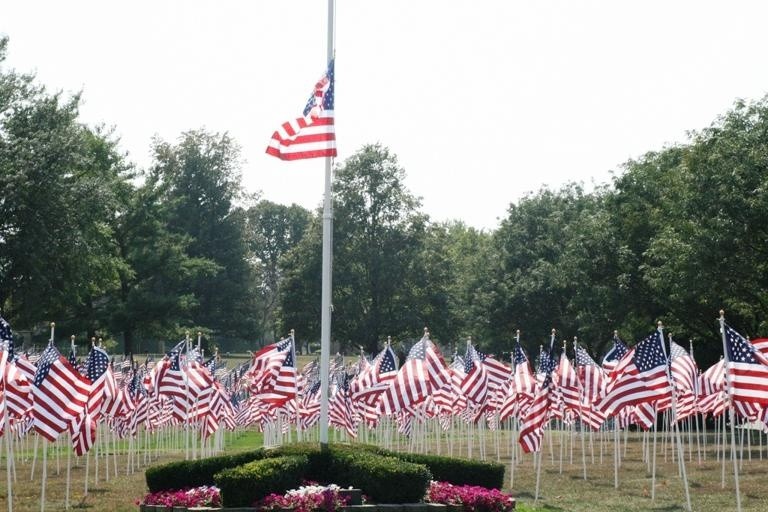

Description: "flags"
0 317 768 457
263 45 341 162
